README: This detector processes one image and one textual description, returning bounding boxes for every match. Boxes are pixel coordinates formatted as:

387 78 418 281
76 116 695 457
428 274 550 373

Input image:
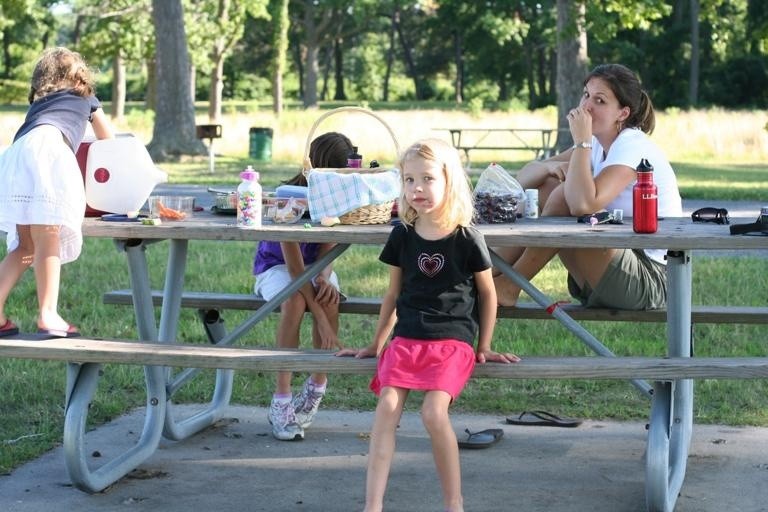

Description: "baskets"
302 106 402 224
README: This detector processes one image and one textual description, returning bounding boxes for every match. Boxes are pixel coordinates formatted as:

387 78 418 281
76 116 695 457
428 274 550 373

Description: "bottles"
523 188 539 220
235 165 263 228
346 146 362 168
760 207 768 223
631 156 658 235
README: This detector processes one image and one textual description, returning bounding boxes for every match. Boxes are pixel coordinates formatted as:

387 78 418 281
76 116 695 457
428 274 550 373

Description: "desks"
82 206 766 512
437 127 572 171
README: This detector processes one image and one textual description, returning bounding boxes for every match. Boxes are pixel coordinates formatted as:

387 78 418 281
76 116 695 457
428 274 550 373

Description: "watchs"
571 141 595 150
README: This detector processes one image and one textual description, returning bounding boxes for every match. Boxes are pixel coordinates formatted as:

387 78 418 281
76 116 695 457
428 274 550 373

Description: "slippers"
457 429 503 449
505 410 582 427
37 325 81 337
0 320 18 337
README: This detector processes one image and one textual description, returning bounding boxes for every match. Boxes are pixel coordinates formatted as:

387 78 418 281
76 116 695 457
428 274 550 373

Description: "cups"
262 203 273 221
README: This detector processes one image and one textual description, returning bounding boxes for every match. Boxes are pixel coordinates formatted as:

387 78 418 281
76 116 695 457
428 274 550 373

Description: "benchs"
100 288 766 464
1 319 766 512
454 145 562 171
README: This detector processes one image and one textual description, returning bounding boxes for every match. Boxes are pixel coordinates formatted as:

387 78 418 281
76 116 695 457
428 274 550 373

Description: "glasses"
691 207 728 225
577 212 613 223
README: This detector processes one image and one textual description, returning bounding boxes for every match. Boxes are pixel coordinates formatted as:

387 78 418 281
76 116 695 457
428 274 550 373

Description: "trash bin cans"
249 128 273 162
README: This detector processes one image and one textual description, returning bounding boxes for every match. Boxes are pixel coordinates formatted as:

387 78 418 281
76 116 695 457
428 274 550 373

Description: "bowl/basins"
146 195 195 220
270 200 306 224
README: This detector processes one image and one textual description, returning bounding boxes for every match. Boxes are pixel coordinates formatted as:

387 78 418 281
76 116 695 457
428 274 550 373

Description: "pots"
204 186 237 211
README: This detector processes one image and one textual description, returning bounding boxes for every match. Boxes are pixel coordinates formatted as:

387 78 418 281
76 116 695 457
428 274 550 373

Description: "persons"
1 46 117 339
353 137 520 512
476 63 686 311
254 129 359 442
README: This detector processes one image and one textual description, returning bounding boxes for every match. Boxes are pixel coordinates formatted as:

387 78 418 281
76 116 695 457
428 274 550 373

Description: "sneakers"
295 377 327 428
266 398 304 441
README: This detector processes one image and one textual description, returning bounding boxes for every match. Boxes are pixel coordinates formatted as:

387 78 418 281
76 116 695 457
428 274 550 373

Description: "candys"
237 189 261 228
